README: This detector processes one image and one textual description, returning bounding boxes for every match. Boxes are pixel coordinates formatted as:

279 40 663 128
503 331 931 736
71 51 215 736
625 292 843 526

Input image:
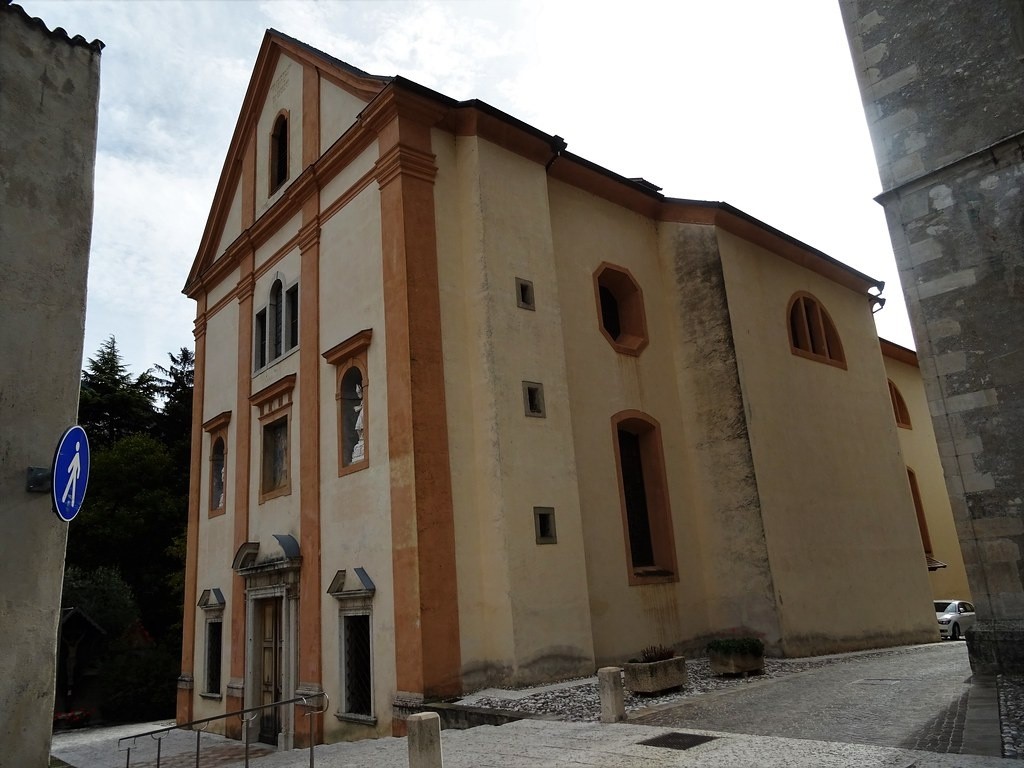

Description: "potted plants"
622 643 687 692
707 637 765 674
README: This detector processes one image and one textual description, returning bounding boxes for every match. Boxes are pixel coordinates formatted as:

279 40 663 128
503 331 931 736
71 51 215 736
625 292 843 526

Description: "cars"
934 599 976 641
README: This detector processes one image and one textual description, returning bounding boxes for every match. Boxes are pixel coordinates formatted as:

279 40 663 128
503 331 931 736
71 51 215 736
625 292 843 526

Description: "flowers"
54 704 96 729
